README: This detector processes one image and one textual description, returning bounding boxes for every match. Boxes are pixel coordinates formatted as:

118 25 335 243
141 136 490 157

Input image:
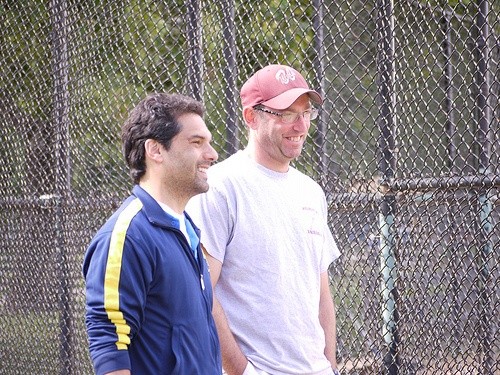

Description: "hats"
240 65 324 110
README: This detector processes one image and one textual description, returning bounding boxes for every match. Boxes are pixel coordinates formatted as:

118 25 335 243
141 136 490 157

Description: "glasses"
252 106 318 123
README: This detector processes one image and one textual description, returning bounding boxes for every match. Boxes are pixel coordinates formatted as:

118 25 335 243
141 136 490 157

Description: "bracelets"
333 367 339 375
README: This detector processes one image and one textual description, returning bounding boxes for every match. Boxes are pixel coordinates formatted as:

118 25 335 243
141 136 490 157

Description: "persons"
184 65 341 375
83 93 223 375
